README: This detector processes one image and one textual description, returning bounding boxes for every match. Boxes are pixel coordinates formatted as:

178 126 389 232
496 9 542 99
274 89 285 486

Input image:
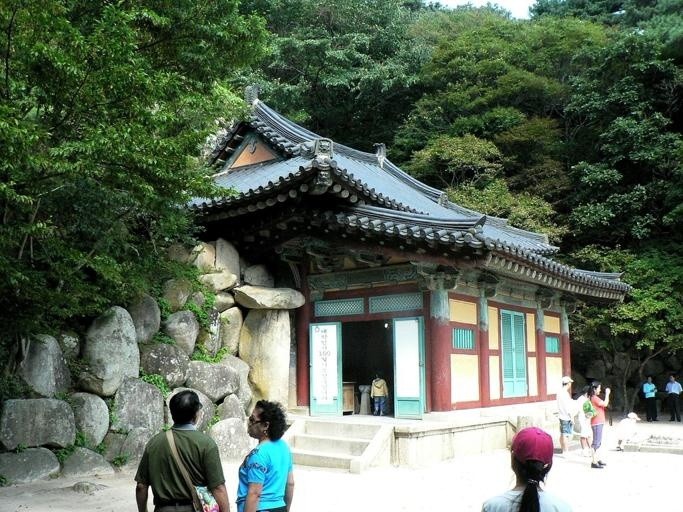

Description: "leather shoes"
591 461 606 468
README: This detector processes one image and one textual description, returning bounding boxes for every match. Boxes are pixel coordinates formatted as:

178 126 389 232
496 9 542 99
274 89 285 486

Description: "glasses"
248 417 262 423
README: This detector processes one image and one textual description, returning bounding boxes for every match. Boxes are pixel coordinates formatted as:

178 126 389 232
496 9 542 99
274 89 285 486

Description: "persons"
642 376 658 422
370 369 389 417
480 427 570 512
135 391 231 512
555 376 610 470
235 399 294 512
663 373 681 423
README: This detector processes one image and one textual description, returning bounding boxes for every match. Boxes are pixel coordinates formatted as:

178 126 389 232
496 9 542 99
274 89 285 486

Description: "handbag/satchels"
192 486 220 512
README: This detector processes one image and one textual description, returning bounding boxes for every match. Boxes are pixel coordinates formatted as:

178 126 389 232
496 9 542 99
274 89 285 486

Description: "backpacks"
583 395 597 418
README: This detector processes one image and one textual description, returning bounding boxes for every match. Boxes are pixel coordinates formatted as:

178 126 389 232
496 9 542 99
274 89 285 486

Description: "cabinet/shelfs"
341 381 356 414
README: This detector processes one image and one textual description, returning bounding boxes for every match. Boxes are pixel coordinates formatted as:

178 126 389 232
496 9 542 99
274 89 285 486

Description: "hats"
562 376 574 384
511 427 553 468
